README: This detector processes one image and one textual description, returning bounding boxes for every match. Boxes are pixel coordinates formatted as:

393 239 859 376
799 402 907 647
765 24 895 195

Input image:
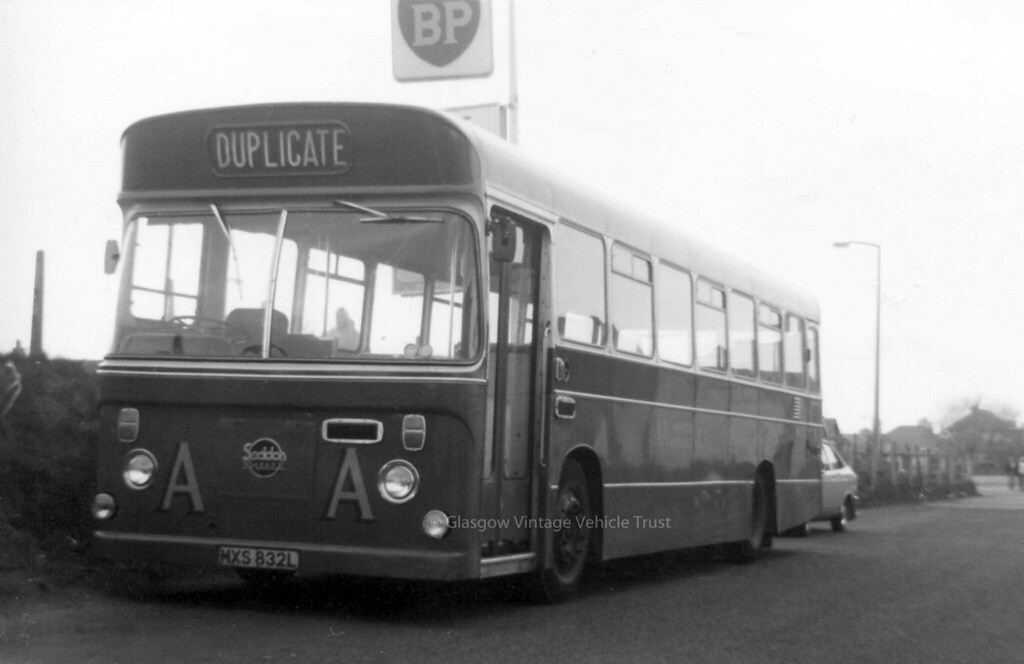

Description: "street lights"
834 241 882 490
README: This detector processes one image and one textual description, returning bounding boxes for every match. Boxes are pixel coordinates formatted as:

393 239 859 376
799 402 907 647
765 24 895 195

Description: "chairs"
220 308 288 358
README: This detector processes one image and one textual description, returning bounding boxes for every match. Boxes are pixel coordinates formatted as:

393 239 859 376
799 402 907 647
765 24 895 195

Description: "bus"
84 102 823 605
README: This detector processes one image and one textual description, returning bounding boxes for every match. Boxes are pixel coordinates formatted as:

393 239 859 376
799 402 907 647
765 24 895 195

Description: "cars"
792 438 859 537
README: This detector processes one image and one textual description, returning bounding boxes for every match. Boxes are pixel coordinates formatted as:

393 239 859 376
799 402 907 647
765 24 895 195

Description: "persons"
1007 456 1024 491
